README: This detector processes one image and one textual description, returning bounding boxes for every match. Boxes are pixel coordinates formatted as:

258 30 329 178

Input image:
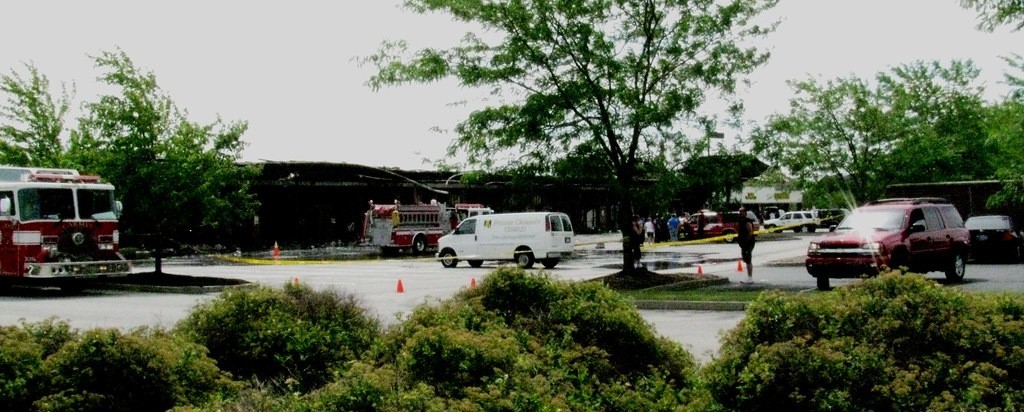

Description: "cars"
965 215 1024 263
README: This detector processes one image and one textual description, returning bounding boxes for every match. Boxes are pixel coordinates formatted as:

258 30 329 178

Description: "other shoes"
740 279 754 283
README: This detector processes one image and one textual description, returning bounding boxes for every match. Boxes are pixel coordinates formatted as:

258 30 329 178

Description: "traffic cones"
697 266 703 276
470 277 476 288
736 259 744 271
272 241 280 256
293 276 299 287
396 278 404 294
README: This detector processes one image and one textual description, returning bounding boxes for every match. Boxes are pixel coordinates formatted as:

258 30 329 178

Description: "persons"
738 207 755 285
645 213 686 245
633 213 644 269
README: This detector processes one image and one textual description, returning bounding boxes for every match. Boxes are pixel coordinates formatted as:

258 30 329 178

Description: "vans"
434 211 574 270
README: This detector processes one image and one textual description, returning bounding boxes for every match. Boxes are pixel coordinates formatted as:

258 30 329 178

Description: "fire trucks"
0 165 132 280
358 198 495 257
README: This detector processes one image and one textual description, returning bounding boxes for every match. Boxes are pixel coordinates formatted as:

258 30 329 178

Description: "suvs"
804 198 972 291
682 210 760 244
763 210 817 234
